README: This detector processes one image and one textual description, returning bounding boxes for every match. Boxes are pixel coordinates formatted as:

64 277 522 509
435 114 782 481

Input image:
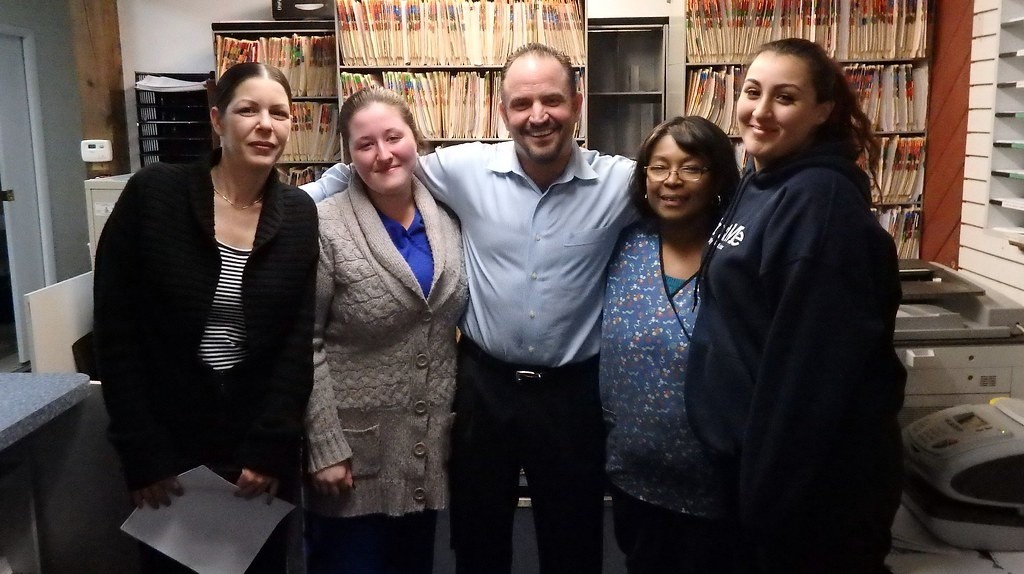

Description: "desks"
0 372 90 574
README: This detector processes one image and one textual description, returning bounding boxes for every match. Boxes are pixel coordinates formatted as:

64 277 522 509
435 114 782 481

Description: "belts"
461 335 599 389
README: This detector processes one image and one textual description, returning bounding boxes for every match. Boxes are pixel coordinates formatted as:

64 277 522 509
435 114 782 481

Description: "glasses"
644 165 716 182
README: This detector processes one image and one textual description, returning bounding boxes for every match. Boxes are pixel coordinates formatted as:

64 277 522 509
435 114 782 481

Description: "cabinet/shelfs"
983 0 1024 249
684 0 934 259
211 20 343 186
333 1 588 154
589 24 669 161
134 71 216 168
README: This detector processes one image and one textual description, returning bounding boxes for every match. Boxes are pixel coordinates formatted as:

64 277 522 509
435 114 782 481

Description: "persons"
94 62 320 574
298 42 641 574
598 114 741 574
689 37 909 574
305 86 470 574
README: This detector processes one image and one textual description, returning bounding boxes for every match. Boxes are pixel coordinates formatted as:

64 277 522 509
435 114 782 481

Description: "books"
683 0 927 259
214 0 585 188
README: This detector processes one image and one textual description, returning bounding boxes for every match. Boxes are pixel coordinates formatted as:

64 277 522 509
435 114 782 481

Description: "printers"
899 396 1024 553
882 257 1024 425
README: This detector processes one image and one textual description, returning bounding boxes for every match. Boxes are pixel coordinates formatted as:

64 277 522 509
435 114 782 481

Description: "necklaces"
214 188 263 210
